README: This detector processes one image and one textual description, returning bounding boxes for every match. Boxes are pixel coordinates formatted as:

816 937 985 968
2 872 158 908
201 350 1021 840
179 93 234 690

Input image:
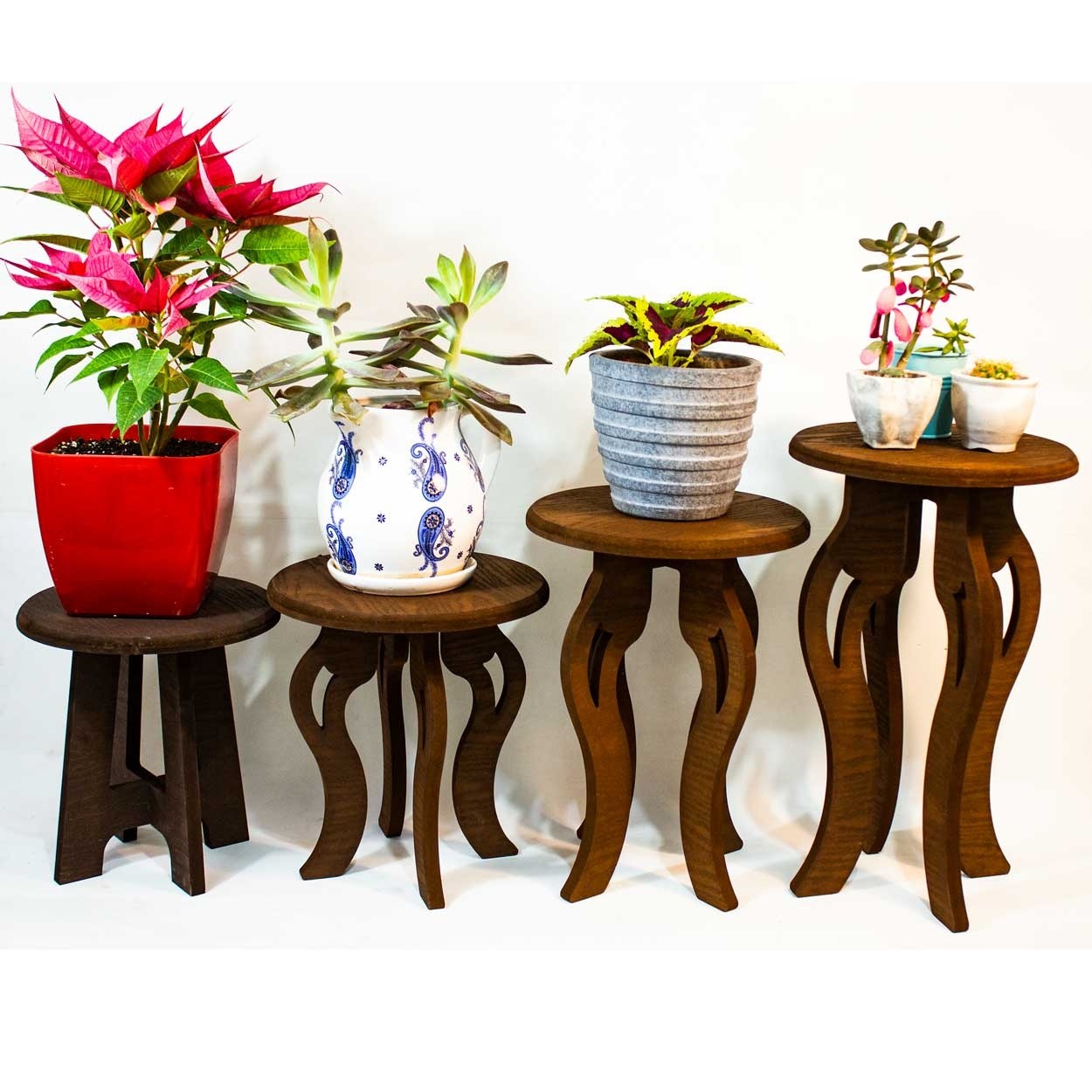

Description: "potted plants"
233 218 553 597
889 316 973 438
847 217 975 447
948 360 1039 455
568 289 785 521
0 84 337 619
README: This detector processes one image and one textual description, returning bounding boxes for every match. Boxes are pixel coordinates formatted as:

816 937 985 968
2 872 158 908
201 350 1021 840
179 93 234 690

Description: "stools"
788 416 1079 931
19 573 282 896
270 550 548 912
531 482 811 915
